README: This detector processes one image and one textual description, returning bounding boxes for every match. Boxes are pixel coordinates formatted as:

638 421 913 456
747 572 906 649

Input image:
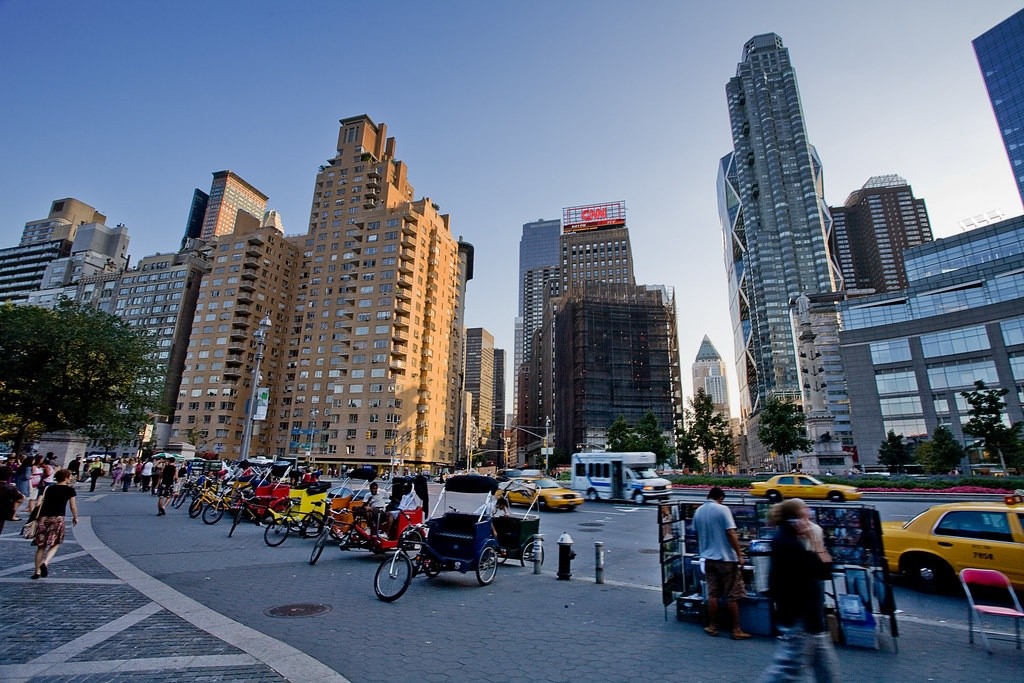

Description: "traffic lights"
416 440 419 445
366 431 370 439
425 429 428 437
404 448 407 453
470 453 472 458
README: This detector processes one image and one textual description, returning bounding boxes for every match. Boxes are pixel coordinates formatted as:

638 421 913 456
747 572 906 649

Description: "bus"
0 453 11 457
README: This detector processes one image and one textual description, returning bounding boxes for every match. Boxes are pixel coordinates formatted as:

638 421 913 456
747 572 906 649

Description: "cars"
748 471 862 506
87 454 111 460
498 469 544 477
882 487 1023 593
433 469 497 483
498 476 585 511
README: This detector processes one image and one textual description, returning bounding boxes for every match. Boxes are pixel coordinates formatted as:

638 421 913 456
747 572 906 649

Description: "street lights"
240 309 272 461
309 407 320 455
147 415 169 422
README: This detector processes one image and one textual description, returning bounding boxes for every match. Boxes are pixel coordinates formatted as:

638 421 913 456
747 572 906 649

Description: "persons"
30 469 79 580
0 451 58 520
67 454 82 487
691 487 751 639
88 456 103 492
155 457 179 516
110 456 191 492
825 468 835 476
487 498 510 538
756 498 849 683
0 466 26 537
362 482 392 538
302 468 324 485
226 460 240 467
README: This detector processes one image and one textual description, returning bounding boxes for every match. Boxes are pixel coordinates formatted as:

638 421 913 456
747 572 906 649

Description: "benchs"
434 513 490 542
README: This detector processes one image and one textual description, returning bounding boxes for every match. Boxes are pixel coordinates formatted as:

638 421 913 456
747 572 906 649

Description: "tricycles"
375 474 545 602
309 474 430 565
172 455 372 547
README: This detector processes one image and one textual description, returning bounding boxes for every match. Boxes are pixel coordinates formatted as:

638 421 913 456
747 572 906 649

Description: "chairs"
959 568 1024 654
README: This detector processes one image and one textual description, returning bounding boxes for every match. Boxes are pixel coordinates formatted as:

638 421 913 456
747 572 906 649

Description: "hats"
76 454 82 458
10 453 16 457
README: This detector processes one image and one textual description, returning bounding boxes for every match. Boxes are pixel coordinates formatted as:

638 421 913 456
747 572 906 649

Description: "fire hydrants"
555 531 577 581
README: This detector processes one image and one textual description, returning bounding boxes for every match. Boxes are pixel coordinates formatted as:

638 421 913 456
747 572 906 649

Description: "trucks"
571 451 674 505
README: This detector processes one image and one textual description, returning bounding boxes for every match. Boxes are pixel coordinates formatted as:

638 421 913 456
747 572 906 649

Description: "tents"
185 457 205 461
150 452 185 460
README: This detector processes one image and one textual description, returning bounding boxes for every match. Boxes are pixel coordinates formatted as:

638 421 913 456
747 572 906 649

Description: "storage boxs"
676 588 707 625
836 609 878 648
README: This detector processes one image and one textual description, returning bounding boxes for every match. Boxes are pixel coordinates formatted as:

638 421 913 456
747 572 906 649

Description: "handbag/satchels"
100 469 103 476
23 521 39 539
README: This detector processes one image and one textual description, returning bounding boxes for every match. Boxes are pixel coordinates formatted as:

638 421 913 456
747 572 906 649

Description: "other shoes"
110 483 157 496
161 506 166 515
8 518 22 521
704 626 719 636
40 562 48 578
156 513 161 516
732 632 751 639
31 574 40 579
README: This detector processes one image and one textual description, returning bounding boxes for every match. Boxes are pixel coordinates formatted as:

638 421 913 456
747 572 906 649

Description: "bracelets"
815 551 828 553
